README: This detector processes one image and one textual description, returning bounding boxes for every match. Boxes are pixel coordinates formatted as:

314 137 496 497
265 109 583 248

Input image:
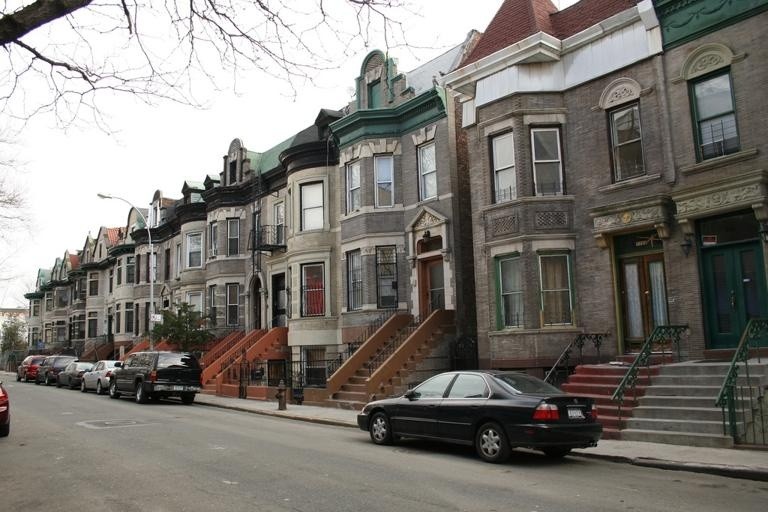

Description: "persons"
36 338 45 349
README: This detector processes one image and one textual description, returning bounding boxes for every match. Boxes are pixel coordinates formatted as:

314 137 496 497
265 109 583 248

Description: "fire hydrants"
275 379 288 411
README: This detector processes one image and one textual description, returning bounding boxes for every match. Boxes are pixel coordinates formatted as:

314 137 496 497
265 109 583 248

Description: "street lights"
96 191 157 352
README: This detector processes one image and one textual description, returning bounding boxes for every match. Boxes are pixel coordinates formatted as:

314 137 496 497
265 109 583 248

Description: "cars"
15 353 125 395
0 378 11 437
357 367 602 466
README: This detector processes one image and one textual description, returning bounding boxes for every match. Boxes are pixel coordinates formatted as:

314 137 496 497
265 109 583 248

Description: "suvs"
107 350 204 406
